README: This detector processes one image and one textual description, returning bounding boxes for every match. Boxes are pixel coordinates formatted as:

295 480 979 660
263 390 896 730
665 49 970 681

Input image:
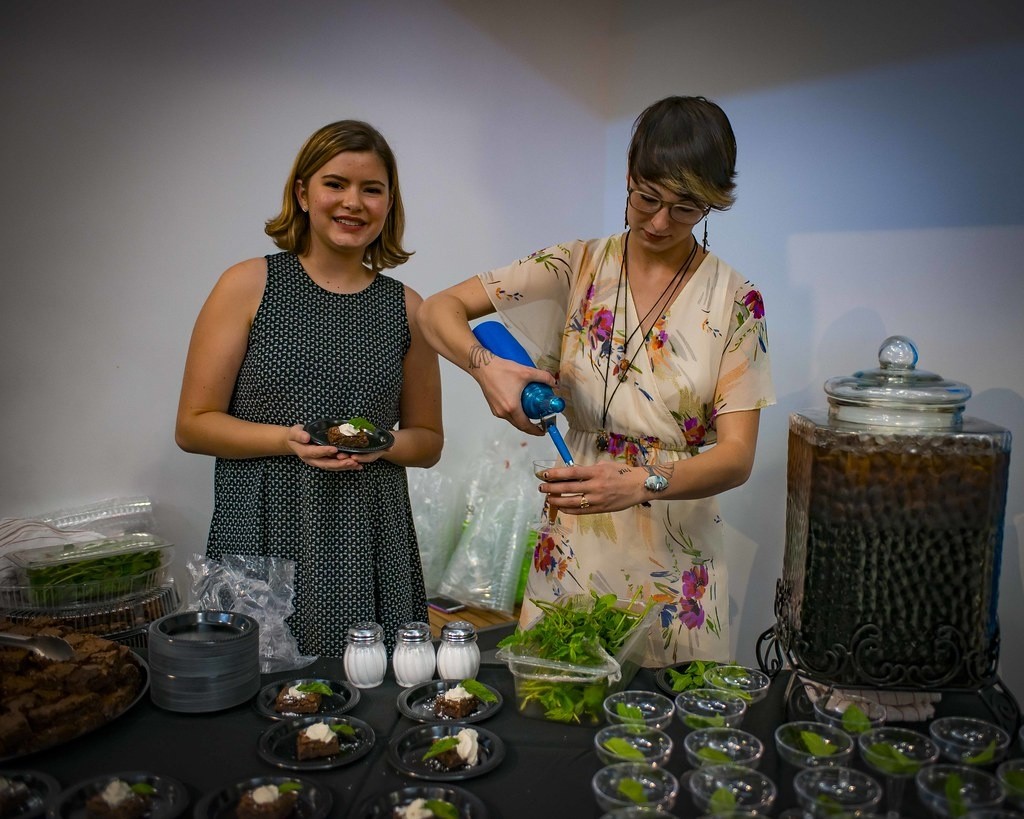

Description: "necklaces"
595 228 699 453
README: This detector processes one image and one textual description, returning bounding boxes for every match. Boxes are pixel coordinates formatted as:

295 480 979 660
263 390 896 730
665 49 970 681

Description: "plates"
303 418 396 453
257 676 362 722
397 677 506 725
112 648 151 719
146 609 263 714
258 715 377 772
195 774 346 819
361 782 493 819
0 769 60 819
54 770 186 819
380 722 505 782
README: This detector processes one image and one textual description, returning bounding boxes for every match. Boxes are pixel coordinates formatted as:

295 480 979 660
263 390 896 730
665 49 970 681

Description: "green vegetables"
497 583 1024 819
461 678 498 702
278 781 304 793
422 737 460 762
349 417 375 431
422 799 460 819
332 725 356 735
297 681 334 696
18 537 167 603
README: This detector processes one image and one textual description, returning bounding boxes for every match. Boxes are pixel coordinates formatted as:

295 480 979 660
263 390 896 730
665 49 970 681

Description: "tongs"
0 632 77 661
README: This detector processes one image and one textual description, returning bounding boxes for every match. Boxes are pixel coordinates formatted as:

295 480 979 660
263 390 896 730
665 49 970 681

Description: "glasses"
627 173 712 225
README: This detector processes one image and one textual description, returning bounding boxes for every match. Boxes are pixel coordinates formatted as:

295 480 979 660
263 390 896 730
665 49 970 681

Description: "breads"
433 685 479 719
0 619 143 758
274 682 323 713
327 423 369 448
295 721 340 761
86 777 160 819
394 795 435 819
233 780 295 819
431 729 479 769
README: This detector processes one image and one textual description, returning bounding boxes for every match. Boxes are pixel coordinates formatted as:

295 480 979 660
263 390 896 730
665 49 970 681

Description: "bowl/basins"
590 664 1024 818
0 534 184 665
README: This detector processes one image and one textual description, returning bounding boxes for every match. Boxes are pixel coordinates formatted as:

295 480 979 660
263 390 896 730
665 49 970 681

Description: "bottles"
342 621 388 689
393 622 436 687
436 621 482 681
821 332 980 434
473 320 565 423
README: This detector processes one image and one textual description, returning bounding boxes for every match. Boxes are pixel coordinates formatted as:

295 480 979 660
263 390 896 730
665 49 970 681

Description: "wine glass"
529 460 583 537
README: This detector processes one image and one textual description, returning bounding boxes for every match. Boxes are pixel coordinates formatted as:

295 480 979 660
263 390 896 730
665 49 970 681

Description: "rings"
580 495 589 509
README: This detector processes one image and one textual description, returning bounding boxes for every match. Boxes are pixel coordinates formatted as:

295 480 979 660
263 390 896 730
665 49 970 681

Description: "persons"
175 120 445 662
414 97 777 668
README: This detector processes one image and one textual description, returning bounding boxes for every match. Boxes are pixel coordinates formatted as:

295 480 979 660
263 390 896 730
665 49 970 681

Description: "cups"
33 493 155 537
410 470 542 617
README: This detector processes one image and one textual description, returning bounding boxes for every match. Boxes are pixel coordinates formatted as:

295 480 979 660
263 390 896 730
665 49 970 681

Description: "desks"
0 654 1024 819
